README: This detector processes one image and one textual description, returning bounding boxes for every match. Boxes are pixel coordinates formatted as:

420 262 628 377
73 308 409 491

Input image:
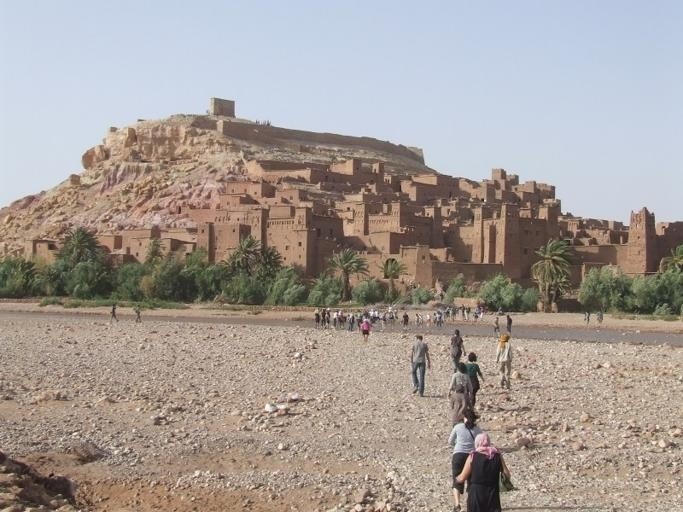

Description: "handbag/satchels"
498 471 513 492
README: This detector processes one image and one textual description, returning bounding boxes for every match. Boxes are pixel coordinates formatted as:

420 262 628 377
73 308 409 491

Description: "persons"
447 409 483 512
596 310 603 323
449 328 467 372
453 432 511 512
110 303 119 321
360 318 372 342
134 304 141 323
310 301 513 337
495 334 512 390
408 334 431 396
445 361 474 402
448 384 470 425
463 352 484 406
584 309 589 323
